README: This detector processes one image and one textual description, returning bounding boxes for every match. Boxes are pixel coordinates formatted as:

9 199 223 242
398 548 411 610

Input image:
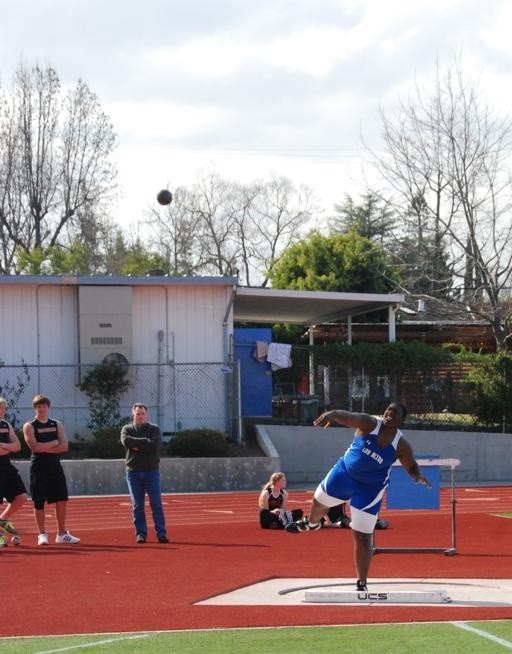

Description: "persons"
257 471 304 530
309 401 431 591
322 503 354 528
0 398 27 550
21 394 81 545
120 402 171 545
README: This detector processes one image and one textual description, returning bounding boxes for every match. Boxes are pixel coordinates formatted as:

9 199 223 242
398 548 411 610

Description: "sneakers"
336 521 341 528
38 532 48 544
56 532 80 544
137 537 145 543
159 537 169 542
286 517 321 533
0 518 18 536
0 535 8 546
357 579 367 591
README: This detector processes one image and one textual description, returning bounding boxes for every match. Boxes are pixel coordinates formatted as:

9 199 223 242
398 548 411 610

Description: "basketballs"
157 190 172 205
